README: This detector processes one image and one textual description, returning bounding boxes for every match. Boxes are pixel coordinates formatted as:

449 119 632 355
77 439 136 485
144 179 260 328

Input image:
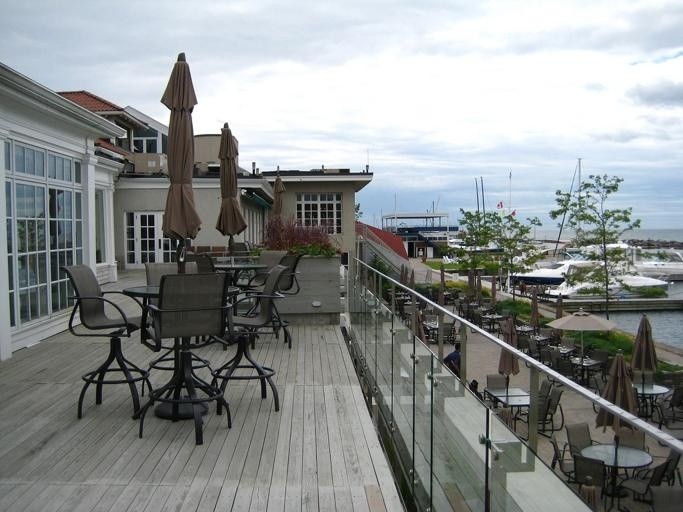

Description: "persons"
520 279 528 294
418 247 426 260
444 340 461 377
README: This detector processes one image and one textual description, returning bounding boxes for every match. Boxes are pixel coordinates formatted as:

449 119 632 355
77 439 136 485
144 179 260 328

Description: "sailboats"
446 157 683 299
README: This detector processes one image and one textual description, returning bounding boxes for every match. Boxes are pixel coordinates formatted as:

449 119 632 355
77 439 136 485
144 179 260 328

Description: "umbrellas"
543 307 618 381
497 312 520 408
530 285 541 336
630 311 659 412
214 122 249 317
398 263 499 316
159 52 204 351
595 347 641 483
273 165 287 222
499 399 515 431
414 307 427 346
